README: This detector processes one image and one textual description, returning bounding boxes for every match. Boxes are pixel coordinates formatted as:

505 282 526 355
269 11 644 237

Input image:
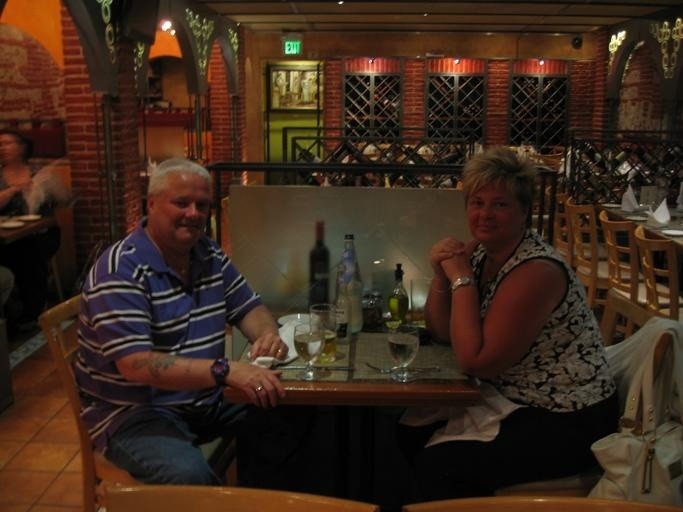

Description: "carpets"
8 310 50 370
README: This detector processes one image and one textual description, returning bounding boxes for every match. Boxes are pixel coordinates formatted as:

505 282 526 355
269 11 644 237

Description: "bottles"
364 83 398 116
359 291 384 330
386 262 409 330
308 221 333 315
333 232 363 334
294 143 471 189
576 136 679 207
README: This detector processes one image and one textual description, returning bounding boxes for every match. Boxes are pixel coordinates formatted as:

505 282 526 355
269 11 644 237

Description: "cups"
309 301 337 363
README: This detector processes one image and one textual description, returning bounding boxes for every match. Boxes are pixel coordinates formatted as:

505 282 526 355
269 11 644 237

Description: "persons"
0 265 15 308
389 146 618 512
0 129 61 330
73 158 304 494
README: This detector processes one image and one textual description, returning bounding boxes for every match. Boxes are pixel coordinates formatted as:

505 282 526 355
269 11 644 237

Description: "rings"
276 349 285 355
254 385 263 392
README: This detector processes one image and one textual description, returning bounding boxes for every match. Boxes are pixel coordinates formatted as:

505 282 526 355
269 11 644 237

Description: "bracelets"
430 285 450 294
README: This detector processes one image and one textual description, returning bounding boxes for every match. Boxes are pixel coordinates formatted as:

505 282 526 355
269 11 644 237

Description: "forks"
365 361 441 373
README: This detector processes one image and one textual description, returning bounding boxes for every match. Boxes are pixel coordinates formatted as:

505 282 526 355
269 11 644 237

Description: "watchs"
210 357 229 387
451 276 476 291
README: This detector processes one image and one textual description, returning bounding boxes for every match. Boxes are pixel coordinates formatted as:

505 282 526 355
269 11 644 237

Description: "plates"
624 214 683 237
278 312 310 326
0 213 42 228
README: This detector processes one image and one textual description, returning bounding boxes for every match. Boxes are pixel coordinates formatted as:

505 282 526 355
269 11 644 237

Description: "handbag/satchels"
588 417 683 507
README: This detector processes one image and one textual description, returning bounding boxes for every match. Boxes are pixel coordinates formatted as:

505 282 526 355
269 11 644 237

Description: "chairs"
402 496 680 512
546 180 683 343
0 317 14 414
492 286 672 493
28 159 75 301
104 483 378 511
37 290 248 512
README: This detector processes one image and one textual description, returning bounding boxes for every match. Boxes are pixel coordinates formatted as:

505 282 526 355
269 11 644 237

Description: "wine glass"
291 321 327 382
385 326 421 382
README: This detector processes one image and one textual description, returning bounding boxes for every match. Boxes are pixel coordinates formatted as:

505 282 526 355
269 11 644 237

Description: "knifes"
276 365 353 372
